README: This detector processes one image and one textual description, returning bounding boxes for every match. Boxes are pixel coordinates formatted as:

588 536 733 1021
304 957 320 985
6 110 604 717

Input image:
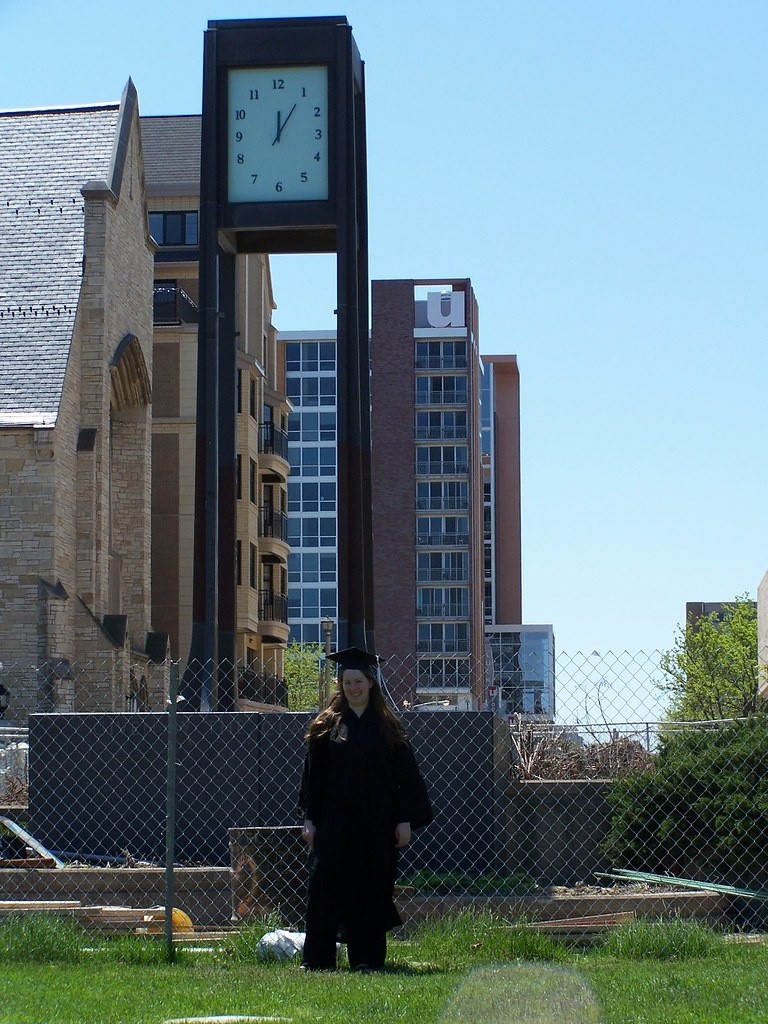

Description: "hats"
325 648 387 687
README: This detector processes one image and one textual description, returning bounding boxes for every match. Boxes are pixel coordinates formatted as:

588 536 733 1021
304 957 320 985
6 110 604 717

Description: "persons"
298 648 433 972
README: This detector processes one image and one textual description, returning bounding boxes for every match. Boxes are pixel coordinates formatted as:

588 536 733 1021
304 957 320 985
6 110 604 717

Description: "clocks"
226 64 328 203
